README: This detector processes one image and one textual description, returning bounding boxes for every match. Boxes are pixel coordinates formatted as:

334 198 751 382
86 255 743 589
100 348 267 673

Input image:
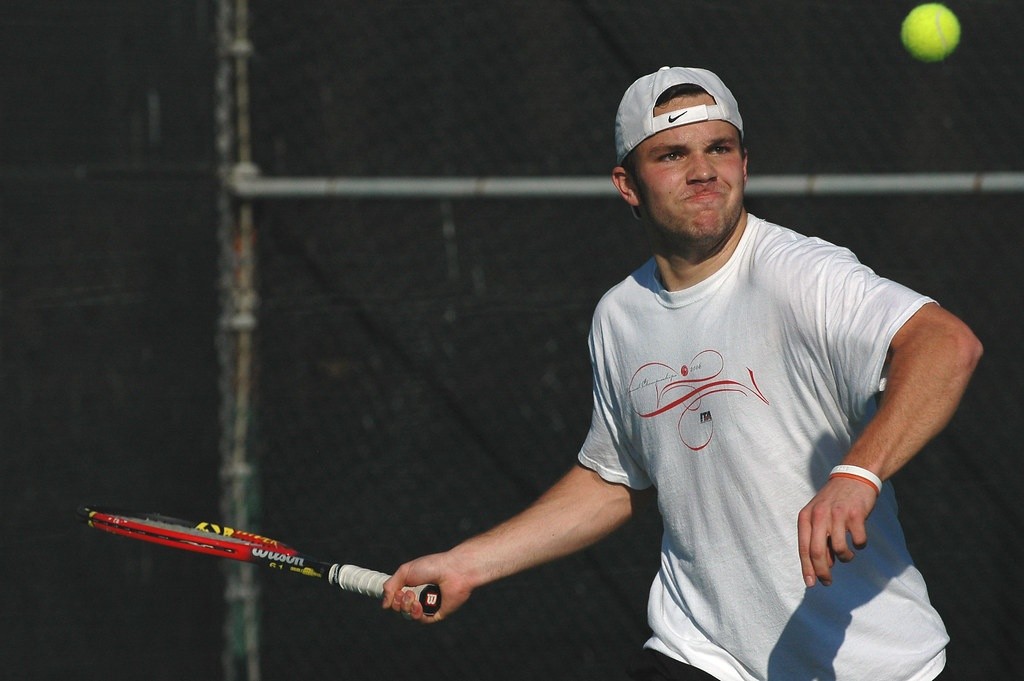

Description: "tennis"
899 2 962 65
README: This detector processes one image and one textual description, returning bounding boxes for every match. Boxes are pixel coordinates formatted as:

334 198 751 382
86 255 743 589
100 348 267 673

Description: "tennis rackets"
81 505 440 618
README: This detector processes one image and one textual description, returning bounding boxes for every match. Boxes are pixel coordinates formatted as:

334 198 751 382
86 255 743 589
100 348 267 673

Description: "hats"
616 66 744 221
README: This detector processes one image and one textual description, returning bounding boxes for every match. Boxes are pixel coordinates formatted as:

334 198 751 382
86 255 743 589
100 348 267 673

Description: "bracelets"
831 465 881 497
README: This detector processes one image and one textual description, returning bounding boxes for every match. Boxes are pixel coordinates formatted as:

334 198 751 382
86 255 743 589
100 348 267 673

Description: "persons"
383 64 984 681
